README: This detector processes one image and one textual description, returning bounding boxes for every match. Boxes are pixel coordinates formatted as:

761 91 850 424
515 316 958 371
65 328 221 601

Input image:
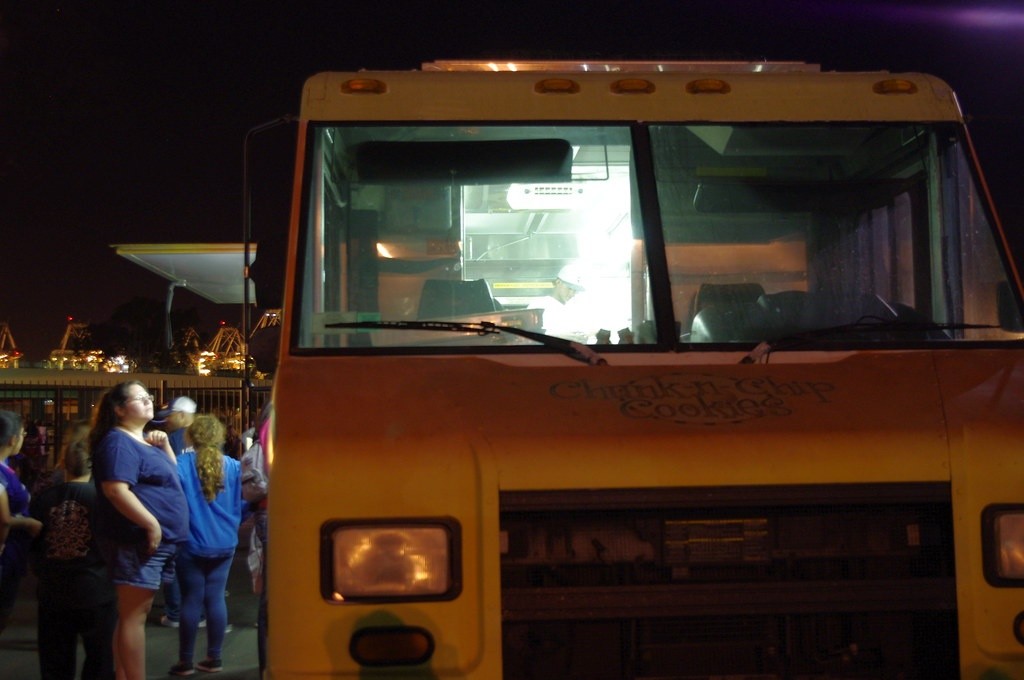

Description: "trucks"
227 60 1024 680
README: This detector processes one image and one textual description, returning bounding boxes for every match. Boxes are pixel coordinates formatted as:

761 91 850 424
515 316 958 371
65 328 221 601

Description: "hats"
150 410 170 424
171 395 197 414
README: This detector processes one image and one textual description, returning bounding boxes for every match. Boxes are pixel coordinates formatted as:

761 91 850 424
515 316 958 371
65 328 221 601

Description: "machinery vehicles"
0 303 281 378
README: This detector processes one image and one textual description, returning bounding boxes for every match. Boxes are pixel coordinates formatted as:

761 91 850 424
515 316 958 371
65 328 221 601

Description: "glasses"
126 395 154 403
20 432 27 437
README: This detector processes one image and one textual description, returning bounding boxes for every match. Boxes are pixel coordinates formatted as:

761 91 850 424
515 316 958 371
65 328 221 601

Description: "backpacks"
241 428 269 500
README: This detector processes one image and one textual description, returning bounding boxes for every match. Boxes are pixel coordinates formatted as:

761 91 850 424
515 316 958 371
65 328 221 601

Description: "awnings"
109 241 257 345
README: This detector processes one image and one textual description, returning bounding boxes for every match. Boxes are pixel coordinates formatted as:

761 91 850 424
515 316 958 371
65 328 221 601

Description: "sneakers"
197 660 223 672
170 663 194 675
161 616 179 627
198 620 206 627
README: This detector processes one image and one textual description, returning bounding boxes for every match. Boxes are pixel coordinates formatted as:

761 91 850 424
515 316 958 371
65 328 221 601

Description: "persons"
0 380 269 680
528 264 588 343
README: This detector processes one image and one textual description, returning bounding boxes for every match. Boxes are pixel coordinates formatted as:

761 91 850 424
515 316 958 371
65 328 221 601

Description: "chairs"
692 281 771 341
417 278 502 327
